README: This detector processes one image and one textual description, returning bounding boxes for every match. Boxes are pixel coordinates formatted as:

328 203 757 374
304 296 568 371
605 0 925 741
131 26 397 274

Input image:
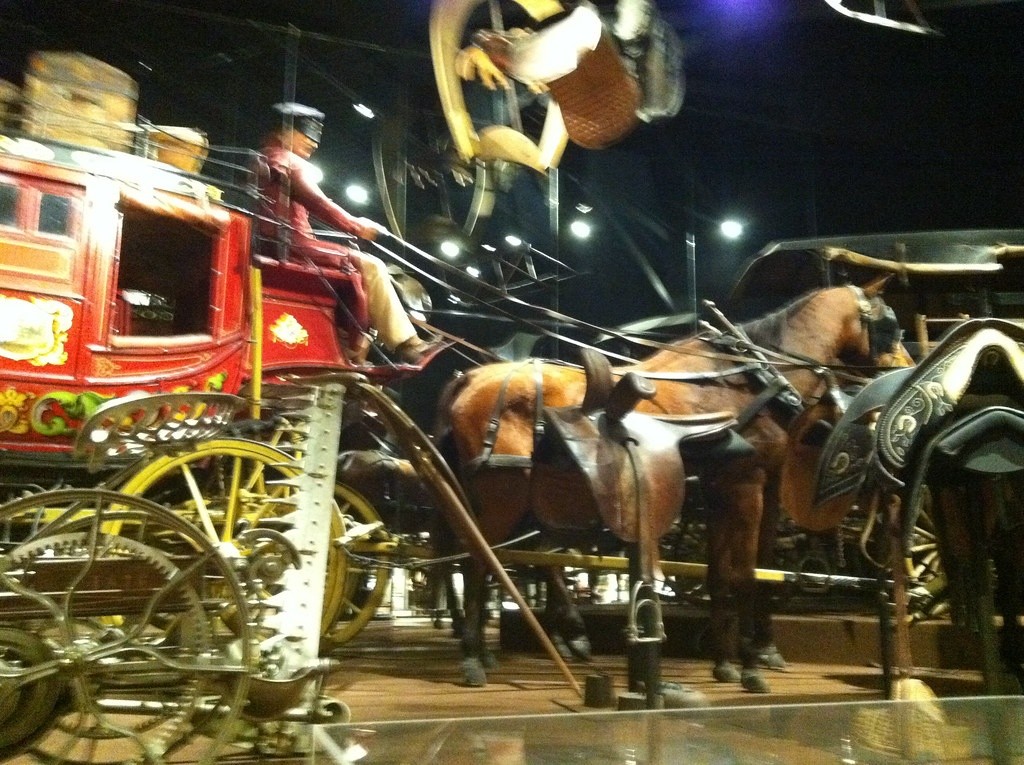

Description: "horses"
434 274 922 709
332 443 592 667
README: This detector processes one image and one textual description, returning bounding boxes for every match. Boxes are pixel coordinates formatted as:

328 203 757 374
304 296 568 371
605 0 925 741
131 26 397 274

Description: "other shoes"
401 333 444 360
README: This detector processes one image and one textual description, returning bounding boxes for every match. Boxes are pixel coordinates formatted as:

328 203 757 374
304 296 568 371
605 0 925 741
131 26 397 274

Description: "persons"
244 103 444 366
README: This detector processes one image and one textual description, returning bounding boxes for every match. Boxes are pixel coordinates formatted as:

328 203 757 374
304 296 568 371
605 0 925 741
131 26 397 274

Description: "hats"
271 101 326 145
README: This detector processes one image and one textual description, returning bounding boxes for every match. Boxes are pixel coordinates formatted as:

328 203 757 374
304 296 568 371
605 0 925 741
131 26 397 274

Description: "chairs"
247 161 360 295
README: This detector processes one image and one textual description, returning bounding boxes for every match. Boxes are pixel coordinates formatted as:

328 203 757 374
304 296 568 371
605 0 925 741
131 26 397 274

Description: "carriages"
0 41 1024 764
